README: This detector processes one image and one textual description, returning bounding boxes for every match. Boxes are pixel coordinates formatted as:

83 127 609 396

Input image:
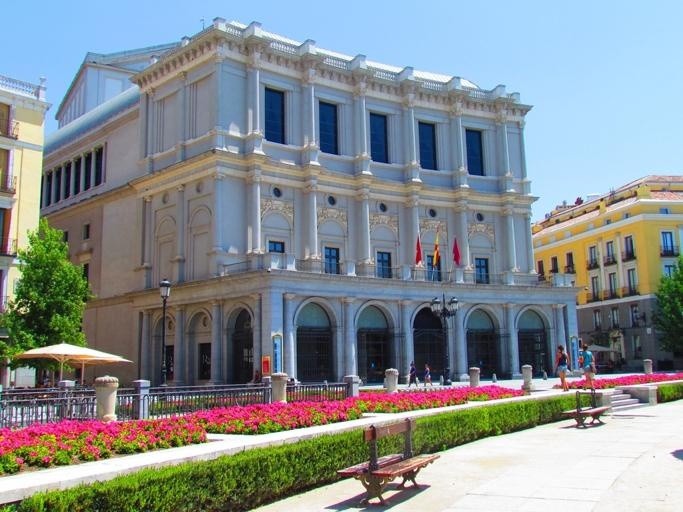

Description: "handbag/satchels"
591 364 597 372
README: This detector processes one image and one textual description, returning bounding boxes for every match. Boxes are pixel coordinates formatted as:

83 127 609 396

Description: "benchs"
337 416 441 507
561 391 611 429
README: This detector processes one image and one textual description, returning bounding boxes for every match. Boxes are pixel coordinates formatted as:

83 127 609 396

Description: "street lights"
159 278 172 401
430 293 459 385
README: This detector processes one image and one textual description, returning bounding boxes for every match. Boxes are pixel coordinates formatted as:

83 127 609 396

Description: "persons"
423 363 433 387
577 344 597 391
555 344 570 392
74 376 79 384
253 370 259 383
44 376 50 387
83 380 88 387
407 363 418 387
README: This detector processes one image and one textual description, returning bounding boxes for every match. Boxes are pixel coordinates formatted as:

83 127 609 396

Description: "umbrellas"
70 346 134 385
12 340 124 381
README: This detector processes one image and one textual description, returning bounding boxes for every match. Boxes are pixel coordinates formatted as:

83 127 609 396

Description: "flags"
415 236 422 265
453 238 461 265
432 234 440 267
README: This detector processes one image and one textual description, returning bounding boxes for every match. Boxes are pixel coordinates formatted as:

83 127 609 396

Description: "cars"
595 361 614 374
